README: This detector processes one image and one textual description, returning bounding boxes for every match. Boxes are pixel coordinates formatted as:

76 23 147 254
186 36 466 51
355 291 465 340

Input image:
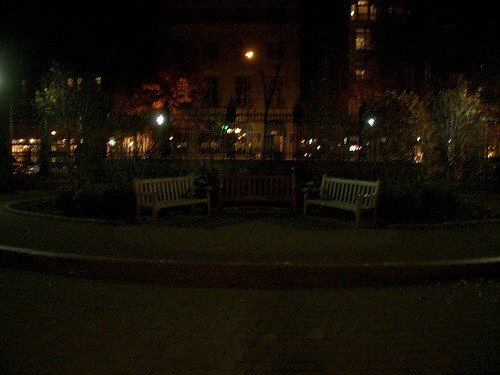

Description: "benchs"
134 173 210 223
303 174 380 226
216 173 296 214
